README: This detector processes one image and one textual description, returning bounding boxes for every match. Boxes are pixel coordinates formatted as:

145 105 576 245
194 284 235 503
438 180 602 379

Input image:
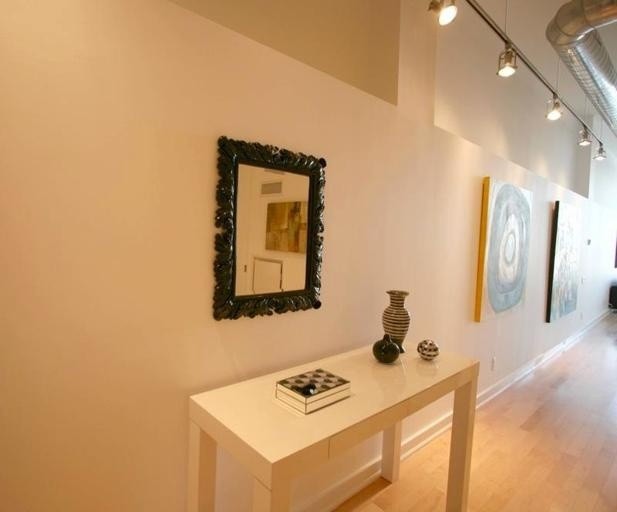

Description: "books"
275 384 351 416
275 367 351 404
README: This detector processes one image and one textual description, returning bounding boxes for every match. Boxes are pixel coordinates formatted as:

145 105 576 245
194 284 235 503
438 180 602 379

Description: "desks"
184 341 481 511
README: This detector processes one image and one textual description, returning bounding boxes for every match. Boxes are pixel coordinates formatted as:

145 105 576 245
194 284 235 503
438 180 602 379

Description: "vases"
382 290 411 353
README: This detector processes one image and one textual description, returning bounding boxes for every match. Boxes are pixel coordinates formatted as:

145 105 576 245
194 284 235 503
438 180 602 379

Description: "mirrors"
211 132 327 321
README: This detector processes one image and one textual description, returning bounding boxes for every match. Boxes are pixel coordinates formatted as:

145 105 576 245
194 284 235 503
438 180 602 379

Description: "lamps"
428 0 606 164
